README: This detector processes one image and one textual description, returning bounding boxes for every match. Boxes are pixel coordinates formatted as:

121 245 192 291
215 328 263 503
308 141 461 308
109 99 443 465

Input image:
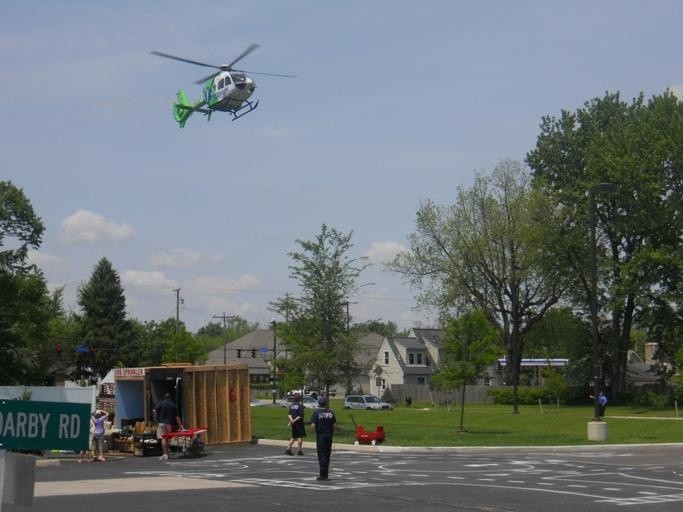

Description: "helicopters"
143 43 298 128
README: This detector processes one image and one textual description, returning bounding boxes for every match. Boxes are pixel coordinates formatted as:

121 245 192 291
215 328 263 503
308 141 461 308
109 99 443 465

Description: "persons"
310 396 343 480
404 393 411 407
283 392 307 455
317 390 324 400
91 410 109 461
88 411 98 459
152 392 184 459
351 383 358 393
588 388 607 416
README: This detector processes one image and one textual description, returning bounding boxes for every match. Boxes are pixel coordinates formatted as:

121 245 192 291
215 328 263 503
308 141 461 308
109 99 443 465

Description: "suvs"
343 393 394 411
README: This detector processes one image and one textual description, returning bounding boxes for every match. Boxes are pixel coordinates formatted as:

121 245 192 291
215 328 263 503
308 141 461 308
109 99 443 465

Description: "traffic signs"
1 400 91 454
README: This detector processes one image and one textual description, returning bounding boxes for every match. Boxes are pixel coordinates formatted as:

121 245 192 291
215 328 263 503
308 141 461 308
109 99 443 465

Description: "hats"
294 393 300 400
97 409 102 415
318 397 327 406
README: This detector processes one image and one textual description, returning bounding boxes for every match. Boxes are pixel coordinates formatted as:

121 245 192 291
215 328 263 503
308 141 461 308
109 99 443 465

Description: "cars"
280 386 335 411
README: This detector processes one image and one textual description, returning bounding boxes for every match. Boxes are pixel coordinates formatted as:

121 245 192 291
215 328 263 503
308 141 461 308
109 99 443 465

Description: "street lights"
586 180 617 421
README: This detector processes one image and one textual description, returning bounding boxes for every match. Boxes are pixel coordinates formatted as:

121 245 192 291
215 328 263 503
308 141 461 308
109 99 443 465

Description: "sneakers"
317 478 328 481
77 456 106 463
285 449 295 455
158 454 169 460
297 451 304 455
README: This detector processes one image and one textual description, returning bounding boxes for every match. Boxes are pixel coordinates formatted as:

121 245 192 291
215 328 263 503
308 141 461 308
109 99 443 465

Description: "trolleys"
349 413 385 447
